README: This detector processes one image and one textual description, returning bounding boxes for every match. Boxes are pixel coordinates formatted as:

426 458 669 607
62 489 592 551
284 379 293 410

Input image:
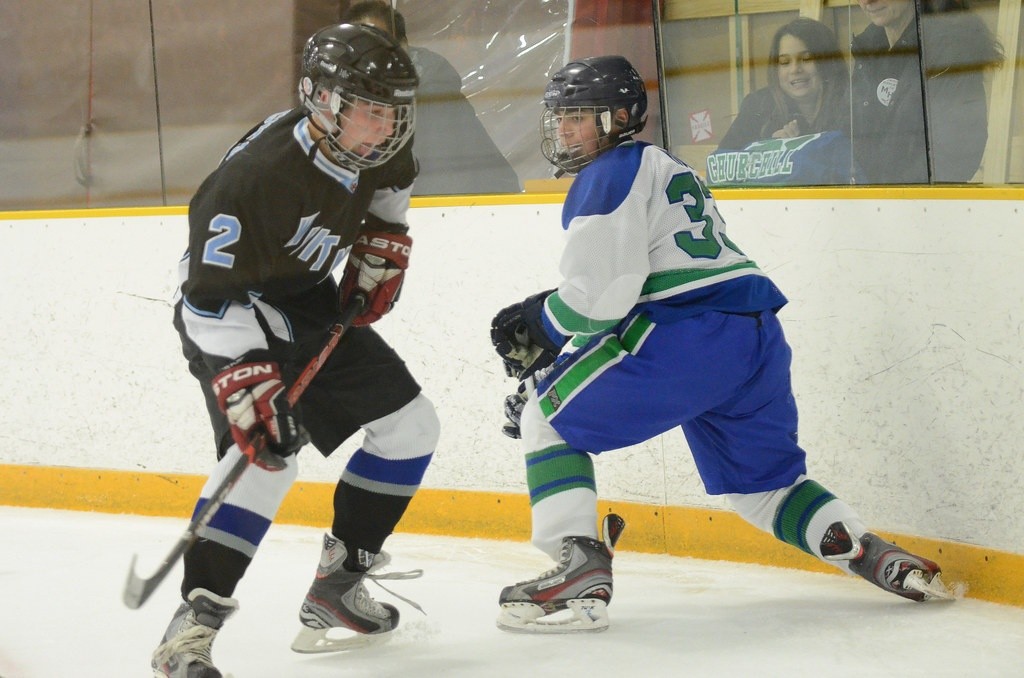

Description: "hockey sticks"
123 290 366 609
514 317 545 404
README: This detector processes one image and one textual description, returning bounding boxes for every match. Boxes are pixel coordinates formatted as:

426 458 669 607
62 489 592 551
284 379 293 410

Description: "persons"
848 0 1005 185
151 23 440 678
707 18 863 185
494 55 964 634
341 0 521 197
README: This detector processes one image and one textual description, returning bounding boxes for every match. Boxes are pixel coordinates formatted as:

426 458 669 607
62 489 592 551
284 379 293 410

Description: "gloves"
490 288 574 382
335 224 413 327
502 352 572 440
210 348 311 472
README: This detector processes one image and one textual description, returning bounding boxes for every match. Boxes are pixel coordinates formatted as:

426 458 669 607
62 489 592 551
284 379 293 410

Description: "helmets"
539 54 648 177
298 22 421 171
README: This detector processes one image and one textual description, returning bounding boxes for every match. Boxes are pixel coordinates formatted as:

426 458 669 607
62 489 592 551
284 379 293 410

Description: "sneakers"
495 513 626 633
290 531 427 653
151 588 239 678
819 520 956 604
